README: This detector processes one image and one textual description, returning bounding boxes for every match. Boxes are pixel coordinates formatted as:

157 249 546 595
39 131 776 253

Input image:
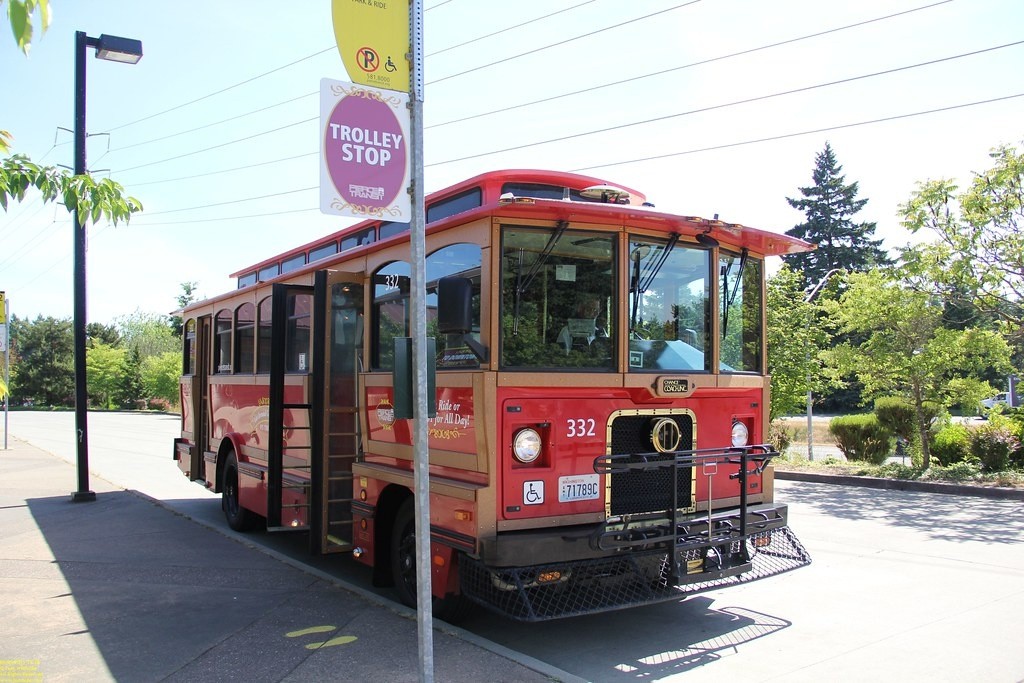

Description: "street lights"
73 31 144 504
803 268 851 461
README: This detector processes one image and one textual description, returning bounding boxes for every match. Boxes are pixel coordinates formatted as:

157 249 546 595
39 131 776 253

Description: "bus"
174 172 818 638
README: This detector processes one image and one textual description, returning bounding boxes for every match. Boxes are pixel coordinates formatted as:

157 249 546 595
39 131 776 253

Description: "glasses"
591 308 603 313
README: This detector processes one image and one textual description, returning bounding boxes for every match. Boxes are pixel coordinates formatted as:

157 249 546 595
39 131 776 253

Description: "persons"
554 289 608 354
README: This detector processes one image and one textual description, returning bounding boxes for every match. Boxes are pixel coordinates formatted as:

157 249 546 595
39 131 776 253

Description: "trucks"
978 392 1024 419
